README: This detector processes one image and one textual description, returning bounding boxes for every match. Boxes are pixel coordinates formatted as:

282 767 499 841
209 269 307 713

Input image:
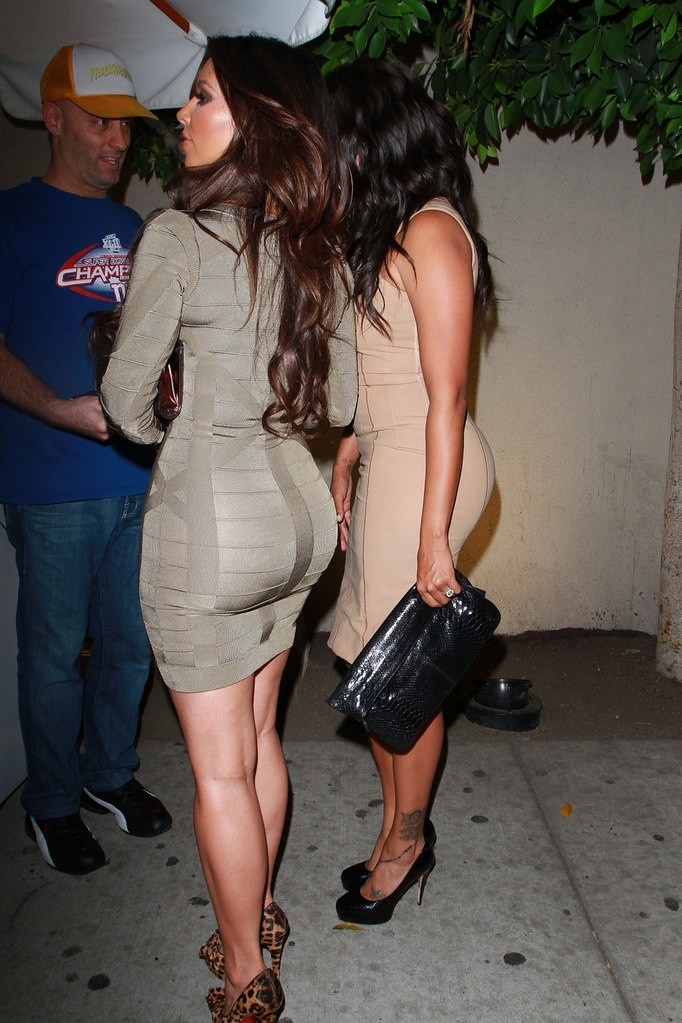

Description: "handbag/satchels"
95 341 185 417
325 567 501 755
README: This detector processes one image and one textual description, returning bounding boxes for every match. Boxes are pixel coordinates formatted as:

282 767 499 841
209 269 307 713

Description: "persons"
1 42 177 879
320 54 506 928
97 30 364 1022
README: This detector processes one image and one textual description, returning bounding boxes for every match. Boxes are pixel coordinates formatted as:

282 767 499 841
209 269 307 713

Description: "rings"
440 586 455 598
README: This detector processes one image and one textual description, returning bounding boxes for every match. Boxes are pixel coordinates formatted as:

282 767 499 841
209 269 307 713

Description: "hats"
40 43 161 120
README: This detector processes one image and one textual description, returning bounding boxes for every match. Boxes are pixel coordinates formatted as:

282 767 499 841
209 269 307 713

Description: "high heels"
336 841 435 927
340 816 436 891
206 972 285 1023
200 903 289 977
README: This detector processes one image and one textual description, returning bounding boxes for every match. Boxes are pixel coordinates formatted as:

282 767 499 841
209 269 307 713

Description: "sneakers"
25 814 106 876
82 778 172 838
